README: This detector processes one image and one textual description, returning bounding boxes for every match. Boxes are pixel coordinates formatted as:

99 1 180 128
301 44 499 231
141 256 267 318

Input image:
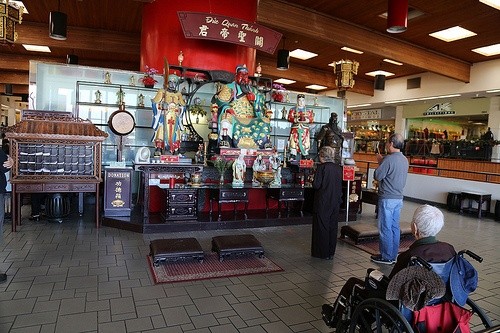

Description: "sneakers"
370 255 396 264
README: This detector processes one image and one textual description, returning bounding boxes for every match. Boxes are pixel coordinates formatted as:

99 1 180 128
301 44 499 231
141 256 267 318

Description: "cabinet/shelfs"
76 80 332 167
348 126 392 154
160 187 198 221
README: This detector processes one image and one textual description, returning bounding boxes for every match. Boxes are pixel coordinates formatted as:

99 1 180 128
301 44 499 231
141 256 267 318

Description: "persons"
370 133 409 265
288 94 314 161
0 147 14 282
212 66 272 149
322 204 456 328
311 146 343 260
316 113 344 164
151 74 186 157
269 151 282 188
232 154 246 188
252 154 266 187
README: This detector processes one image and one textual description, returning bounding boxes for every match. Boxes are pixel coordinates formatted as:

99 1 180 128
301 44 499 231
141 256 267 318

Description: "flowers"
272 84 286 92
144 65 159 77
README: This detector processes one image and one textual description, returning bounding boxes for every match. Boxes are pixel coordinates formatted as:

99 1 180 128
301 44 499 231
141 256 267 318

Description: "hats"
386 265 446 312
450 256 478 307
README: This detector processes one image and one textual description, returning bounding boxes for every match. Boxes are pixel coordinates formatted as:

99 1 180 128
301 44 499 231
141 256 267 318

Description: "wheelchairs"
320 250 494 333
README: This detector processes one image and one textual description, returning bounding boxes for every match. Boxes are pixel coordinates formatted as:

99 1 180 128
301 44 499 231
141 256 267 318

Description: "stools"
447 191 500 222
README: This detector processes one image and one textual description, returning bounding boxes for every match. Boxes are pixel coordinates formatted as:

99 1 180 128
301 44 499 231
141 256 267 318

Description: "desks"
131 164 203 223
358 189 380 220
207 186 249 221
456 191 492 219
265 187 304 219
217 147 272 170
149 221 414 266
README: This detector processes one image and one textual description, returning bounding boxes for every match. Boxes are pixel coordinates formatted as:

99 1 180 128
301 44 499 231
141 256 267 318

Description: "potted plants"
208 156 233 185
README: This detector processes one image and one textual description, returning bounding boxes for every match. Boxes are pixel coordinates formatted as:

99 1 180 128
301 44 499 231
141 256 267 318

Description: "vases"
272 93 284 102
141 77 155 87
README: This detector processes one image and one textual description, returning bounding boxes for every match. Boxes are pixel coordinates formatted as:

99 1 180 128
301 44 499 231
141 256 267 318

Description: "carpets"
339 236 420 256
147 252 286 285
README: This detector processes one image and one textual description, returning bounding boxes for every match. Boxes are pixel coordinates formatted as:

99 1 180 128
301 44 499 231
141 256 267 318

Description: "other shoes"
322 304 334 315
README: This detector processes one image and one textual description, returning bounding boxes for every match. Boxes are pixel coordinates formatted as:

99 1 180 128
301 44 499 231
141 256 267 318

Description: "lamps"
49 0 67 40
276 0 407 91
